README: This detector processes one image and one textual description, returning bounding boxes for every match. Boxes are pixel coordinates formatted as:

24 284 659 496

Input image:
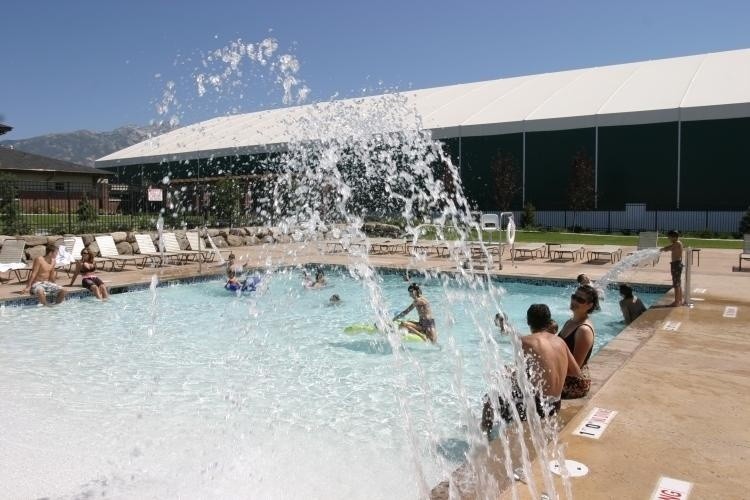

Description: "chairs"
470 244 506 256
0 226 295 284
326 244 378 253
378 242 405 253
434 245 465 257
553 243 586 263
586 248 623 264
626 232 662 267
406 246 434 257
508 242 547 260
738 234 750 270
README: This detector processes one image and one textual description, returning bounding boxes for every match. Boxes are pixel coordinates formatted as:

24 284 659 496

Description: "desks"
545 243 562 257
692 248 701 266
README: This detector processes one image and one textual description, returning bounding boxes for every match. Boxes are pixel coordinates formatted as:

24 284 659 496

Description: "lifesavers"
344 319 432 342
506 220 516 243
224 277 259 292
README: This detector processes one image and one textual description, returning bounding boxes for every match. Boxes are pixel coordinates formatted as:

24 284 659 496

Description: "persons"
225 251 264 293
660 230 685 307
619 284 646 325
21 245 67 307
481 303 581 443
313 268 327 288
328 294 341 305
392 283 437 346
557 285 595 400
548 320 559 333
494 311 519 336
68 247 109 302
578 274 604 314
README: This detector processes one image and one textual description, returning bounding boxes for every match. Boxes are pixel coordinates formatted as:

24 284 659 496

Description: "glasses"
571 294 589 305
81 253 88 255
